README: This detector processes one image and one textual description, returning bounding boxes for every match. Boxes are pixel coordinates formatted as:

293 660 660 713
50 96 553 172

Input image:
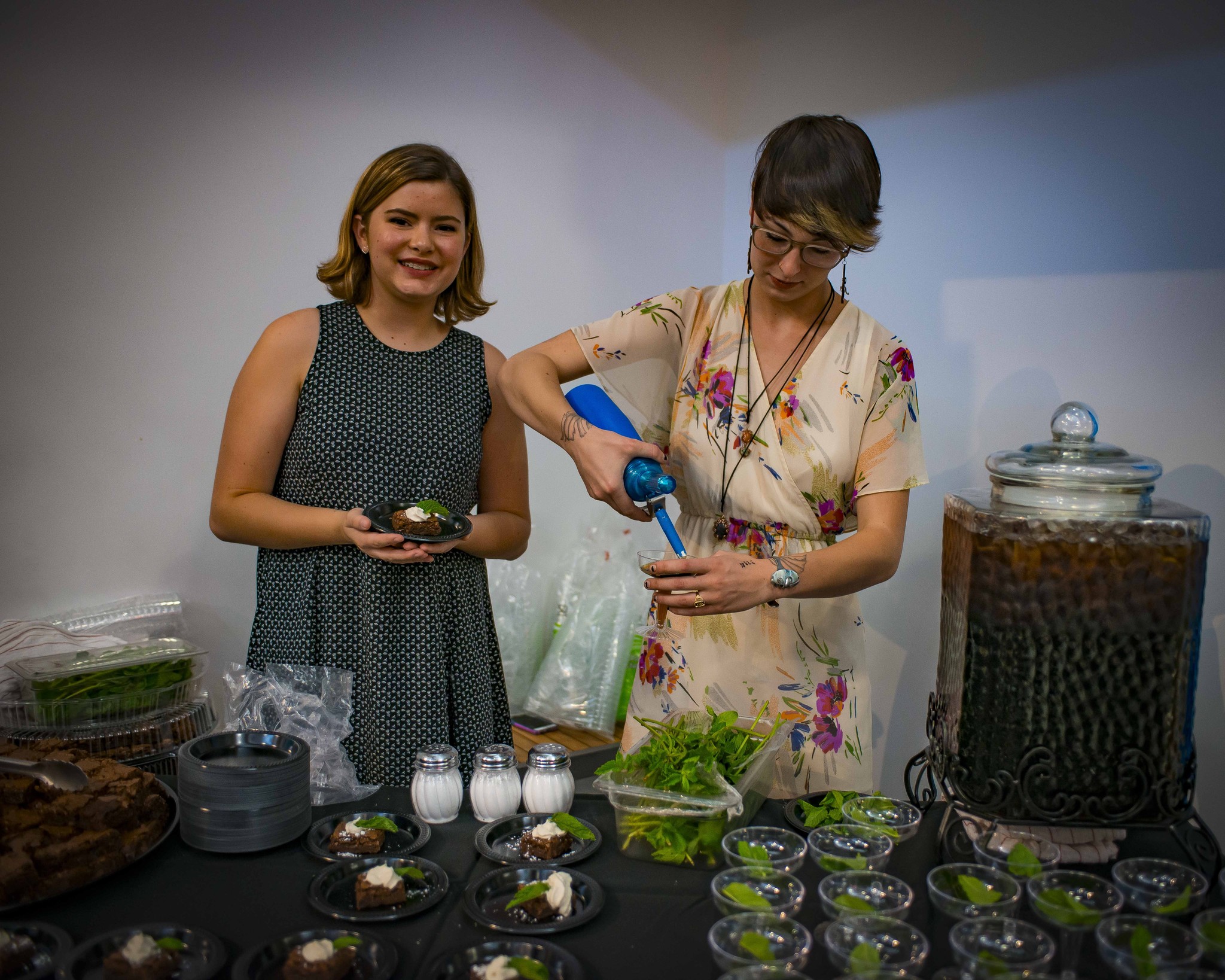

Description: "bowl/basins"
699 797 1225 980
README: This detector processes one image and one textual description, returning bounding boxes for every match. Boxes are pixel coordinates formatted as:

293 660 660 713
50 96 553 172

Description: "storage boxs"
593 713 790 867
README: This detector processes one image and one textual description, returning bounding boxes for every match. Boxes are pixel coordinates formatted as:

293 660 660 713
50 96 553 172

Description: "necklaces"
713 274 835 542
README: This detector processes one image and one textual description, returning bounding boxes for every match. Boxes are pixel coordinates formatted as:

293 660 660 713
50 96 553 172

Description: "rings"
694 592 705 608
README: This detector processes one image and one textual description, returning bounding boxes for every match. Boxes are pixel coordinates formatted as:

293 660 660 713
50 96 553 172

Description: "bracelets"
767 556 800 607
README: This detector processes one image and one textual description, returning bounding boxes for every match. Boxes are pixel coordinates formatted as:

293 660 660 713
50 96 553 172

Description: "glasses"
750 204 851 269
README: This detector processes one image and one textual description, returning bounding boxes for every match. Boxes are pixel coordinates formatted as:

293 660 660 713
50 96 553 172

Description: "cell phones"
509 715 559 734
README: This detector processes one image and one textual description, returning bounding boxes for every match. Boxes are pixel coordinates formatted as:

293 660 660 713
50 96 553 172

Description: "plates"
229 925 400 980
473 812 602 867
176 729 312 854
432 938 588 980
55 923 228 980
461 864 606 935
362 500 473 542
783 790 873 837
301 809 432 863
0 919 75 980
307 853 449 922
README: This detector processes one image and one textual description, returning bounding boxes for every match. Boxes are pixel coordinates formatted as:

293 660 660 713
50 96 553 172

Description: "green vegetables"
594 700 788 865
721 789 1225 980
30 643 197 727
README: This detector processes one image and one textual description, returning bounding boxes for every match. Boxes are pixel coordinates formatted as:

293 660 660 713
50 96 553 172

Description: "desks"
512 721 628 785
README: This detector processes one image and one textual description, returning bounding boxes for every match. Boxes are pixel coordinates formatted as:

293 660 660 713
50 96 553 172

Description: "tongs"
0 755 89 792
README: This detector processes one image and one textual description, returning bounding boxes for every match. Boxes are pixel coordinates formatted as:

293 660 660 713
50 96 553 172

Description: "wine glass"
634 552 696 640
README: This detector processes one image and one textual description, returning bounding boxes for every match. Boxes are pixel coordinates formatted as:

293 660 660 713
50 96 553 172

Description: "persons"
208 143 531 787
494 115 931 798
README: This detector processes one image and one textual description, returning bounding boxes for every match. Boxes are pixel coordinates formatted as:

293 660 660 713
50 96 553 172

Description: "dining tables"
0 777 480 980
413 795 1225 980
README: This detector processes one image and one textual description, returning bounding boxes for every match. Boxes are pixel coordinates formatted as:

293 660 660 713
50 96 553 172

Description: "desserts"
356 863 425 910
283 934 362 980
469 954 550 980
328 816 399 854
102 932 190 980
519 812 596 858
392 500 449 536
0 701 215 908
505 871 577 919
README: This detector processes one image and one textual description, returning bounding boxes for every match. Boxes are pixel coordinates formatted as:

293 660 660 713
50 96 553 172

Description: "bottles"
469 744 522 824
933 407 1214 825
411 744 464 824
566 385 690 559
523 743 575 815
